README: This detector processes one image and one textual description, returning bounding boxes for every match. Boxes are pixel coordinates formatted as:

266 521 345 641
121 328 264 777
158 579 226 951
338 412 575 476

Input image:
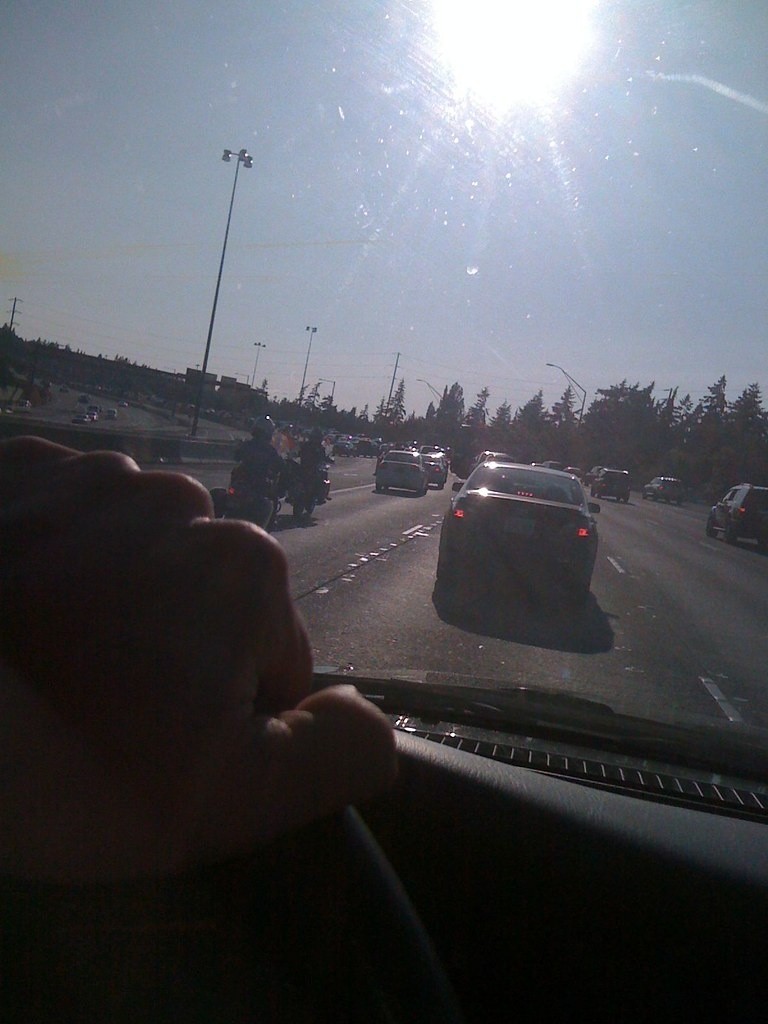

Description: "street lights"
317 376 336 398
234 373 249 384
293 325 318 431
251 341 267 386
546 362 587 436
188 148 254 438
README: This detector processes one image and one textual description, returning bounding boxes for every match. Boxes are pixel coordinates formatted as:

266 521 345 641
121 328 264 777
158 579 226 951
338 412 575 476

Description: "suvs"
590 468 630 504
706 483 768 551
642 476 684 506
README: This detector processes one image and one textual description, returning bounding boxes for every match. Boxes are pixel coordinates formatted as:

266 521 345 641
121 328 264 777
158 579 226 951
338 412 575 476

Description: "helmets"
252 417 275 438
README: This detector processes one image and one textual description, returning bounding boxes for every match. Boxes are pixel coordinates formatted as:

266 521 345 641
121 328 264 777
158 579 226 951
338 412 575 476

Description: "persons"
231 414 283 530
0 436 400 885
301 427 335 505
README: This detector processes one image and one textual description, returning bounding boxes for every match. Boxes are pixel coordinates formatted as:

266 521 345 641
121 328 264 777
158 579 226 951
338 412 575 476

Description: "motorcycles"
285 453 337 519
209 460 291 531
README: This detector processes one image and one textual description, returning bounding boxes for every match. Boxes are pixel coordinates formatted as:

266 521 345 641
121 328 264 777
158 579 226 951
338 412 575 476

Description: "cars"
438 462 602 606
324 429 449 496
465 450 515 476
17 398 32 409
532 461 603 487
42 378 129 426
